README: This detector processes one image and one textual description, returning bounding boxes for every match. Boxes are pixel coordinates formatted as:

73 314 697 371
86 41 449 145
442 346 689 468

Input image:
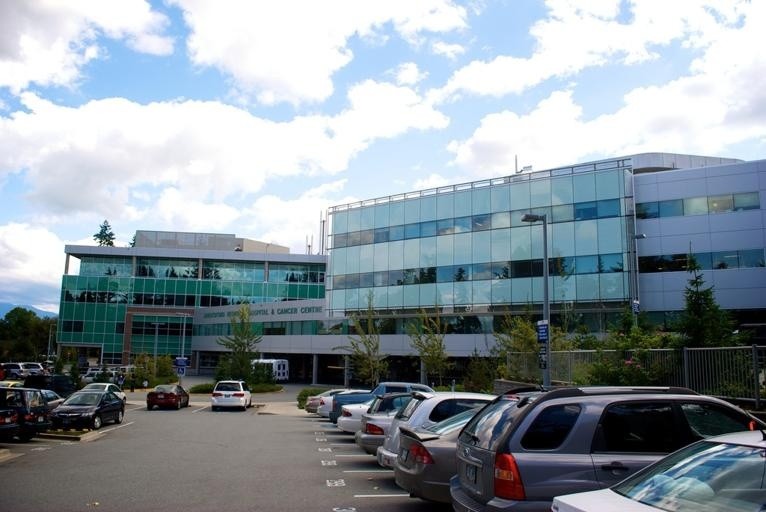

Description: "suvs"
447 384 766 512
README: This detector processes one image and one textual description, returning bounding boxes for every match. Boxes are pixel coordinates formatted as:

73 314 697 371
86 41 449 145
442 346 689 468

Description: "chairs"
88 396 95 403
596 412 687 451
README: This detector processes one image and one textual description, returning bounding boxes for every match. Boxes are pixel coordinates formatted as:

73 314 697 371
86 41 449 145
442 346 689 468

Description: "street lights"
175 312 190 386
144 357 149 371
47 324 56 360
520 213 551 388
151 323 166 358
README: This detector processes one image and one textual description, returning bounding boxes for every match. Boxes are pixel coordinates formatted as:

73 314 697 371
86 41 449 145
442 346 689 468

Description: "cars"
549 428 766 512
392 405 499 512
317 382 435 434
303 388 348 413
0 360 135 440
376 390 498 469
211 379 252 412
355 410 398 456
146 384 189 409
738 323 766 367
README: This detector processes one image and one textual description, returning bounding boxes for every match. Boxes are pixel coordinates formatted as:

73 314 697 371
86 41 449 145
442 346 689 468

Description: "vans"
252 359 289 381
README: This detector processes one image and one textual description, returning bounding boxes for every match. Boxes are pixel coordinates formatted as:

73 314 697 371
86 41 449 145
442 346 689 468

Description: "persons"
118 370 136 392
142 378 149 392
